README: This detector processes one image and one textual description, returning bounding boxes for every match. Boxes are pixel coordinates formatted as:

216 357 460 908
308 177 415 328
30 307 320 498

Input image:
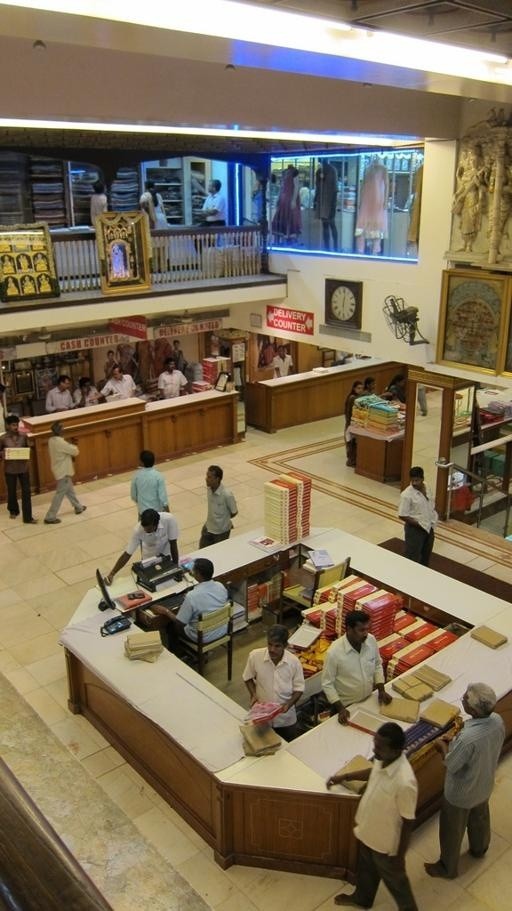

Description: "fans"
383 294 429 346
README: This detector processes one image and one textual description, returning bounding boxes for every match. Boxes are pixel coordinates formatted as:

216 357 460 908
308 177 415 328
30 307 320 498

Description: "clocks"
324 277 362 329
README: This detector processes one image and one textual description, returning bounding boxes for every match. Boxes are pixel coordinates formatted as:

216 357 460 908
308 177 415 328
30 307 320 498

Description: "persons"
326 722 420 910
43 422 86 524
298 182 309 208
258 340 297 378
408 166 423 257
90 181 108 226
0 416 37 524
139 181 169 273
344 374 406 467
250 180 264 252
335 173 347 206
243 623 306 742
151 559 229 664
130 450 170 555
103 507 183 586
313 157 338 251
321 609 392 725
425 683 507 879
201 180 227 247
199 465 238 548
270 174 280 209
272 165 304 243
44 338 193 414
417 382 428 416
353 159 390 253
398 467 437 565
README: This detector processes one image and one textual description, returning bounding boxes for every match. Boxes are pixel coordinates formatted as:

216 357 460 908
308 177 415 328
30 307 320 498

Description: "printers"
132 553 182 592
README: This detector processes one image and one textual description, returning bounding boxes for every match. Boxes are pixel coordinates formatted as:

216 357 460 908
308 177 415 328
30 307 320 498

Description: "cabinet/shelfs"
350 429 406 484
0 150 212 230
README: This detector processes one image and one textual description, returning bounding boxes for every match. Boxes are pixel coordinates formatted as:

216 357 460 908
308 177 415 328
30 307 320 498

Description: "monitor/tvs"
96 569 115 611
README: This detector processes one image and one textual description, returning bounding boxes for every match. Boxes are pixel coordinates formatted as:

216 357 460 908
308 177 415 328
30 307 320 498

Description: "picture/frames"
96 210 151 297
435 269 509 377
500 276 512 381
0 221 60 303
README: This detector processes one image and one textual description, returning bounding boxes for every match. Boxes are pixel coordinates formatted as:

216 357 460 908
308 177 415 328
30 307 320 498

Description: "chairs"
179 601 234 680
279 557 351 627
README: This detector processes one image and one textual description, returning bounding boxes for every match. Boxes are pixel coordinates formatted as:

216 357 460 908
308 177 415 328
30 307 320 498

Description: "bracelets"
164 609 169 617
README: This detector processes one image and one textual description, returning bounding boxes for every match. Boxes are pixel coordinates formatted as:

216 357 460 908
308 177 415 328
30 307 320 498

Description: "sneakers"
76 506 87 515
44 518 61 524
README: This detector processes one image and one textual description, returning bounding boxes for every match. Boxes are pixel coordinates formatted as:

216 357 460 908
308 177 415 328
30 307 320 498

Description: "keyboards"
154 596 185 610
